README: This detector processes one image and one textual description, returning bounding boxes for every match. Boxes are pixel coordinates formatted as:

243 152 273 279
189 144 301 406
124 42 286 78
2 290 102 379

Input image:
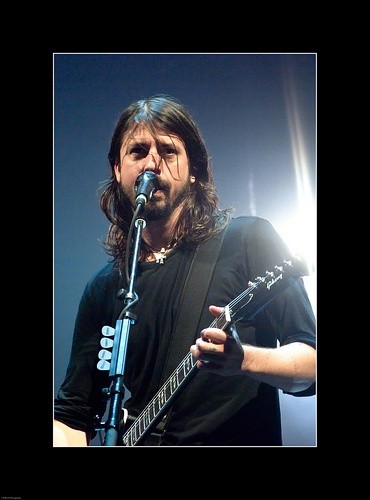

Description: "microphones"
135 171 160 207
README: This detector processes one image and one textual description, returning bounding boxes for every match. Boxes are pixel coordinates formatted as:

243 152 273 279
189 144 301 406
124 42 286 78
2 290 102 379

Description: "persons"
53 98 317 447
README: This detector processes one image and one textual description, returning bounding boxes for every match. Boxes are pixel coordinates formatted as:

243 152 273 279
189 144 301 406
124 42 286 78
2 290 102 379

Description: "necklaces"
143 231 183 264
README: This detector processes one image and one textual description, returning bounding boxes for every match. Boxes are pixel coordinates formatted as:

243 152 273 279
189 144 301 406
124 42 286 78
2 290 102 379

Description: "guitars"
112 254 312 447
